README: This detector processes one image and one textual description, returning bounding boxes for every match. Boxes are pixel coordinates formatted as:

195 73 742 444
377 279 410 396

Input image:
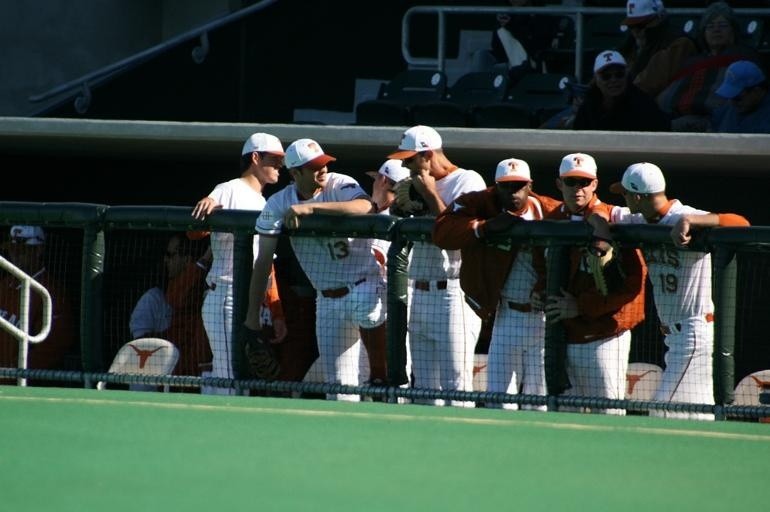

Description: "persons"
431 158 569 413
480 0 769 131
587 160 751 425
530 152 647 414
0 210 215 393
184 129 288 398
243 134 386 403
363 153 415 407
388 120 488 411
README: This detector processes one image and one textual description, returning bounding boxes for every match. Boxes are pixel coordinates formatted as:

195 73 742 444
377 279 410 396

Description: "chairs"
352 66 575 130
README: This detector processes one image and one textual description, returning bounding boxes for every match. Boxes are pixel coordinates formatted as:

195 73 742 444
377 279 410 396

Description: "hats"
11 227 44 246
495 159 531 182
365 125 441 183
241 133 335 170
716 61 764 99
558 153 597 178
610 162 666 194
593 51 626 72
625 1 664 25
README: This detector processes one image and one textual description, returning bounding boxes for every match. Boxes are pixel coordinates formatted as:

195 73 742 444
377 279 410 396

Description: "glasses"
599 70 624 79
564 178 592 187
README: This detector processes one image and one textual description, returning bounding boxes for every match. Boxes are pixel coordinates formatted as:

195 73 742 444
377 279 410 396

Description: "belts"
415 281 446 290
321 280 364 297
660 323 682 335
509 301 532 311
